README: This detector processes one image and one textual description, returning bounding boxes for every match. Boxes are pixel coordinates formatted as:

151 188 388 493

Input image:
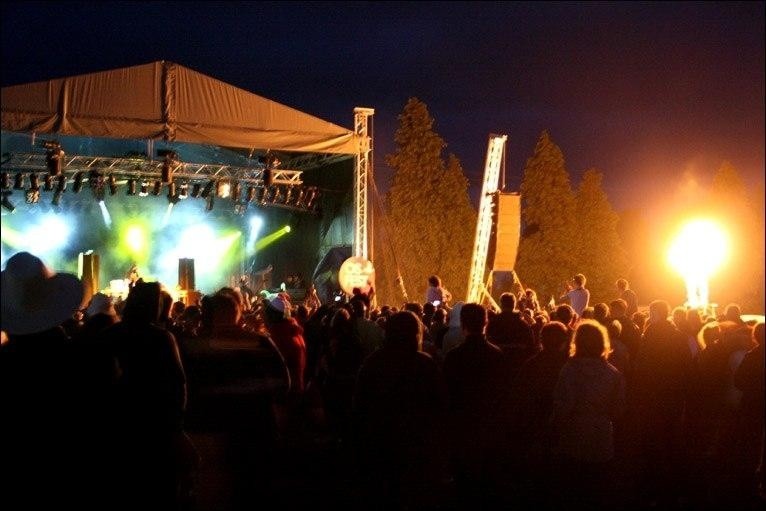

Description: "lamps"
1 141 319 213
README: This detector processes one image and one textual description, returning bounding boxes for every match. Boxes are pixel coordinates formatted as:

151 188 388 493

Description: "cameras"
334 295 342 302
431 300 441 307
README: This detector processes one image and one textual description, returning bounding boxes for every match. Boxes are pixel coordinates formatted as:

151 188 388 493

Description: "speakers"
179 258 194 295
487 190 521 272
77 252 100 298
